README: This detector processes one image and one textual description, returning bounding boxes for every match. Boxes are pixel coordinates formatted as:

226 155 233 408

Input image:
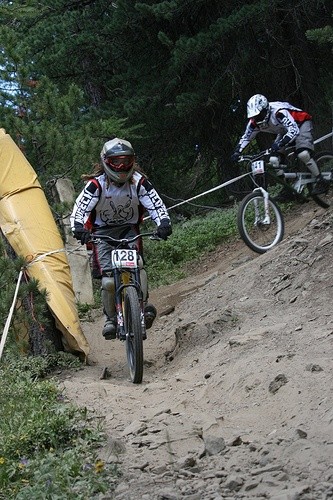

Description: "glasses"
106 156 133 172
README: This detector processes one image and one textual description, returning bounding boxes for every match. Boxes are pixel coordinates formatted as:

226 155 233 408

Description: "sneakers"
273 185 296 203
143 304 157 329
311 176 329 195
102 317 117 340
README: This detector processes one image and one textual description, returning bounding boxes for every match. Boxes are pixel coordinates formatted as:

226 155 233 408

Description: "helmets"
247 94 270 129
100 138 135 183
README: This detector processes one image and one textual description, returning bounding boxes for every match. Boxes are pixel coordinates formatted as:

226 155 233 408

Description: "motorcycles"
232 143 333 254
70 227 170 383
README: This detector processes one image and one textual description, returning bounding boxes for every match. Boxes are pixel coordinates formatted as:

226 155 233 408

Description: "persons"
229 93 331 196
69 138 172 340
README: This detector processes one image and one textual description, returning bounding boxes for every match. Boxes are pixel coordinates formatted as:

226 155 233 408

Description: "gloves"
157 225 172 240
74 228 90 245
271 141 284 151
231 154 241 162
